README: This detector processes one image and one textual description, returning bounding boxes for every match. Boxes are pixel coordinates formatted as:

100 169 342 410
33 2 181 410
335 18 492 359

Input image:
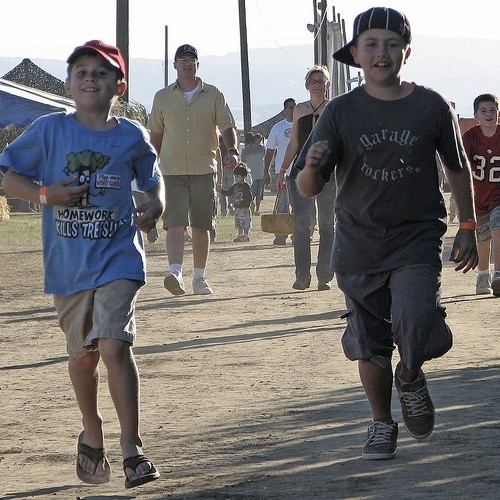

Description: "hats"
305 65 329 82
331 6 412 68
66 40 126 80
175 44 198 62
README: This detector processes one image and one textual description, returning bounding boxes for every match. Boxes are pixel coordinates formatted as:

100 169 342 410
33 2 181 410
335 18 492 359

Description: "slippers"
122 455 161 488
75 430 112 484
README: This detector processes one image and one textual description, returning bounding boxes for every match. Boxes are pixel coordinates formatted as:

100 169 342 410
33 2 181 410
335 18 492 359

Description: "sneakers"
475 273 492 294
362 418 399 460
491 272 500 296
394 361 436 439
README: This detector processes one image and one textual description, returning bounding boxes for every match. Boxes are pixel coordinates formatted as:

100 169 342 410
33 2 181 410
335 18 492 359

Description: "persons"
432 148 459 224
0 41 160 489
142 43 240 294
176 129 285 244
291 7 482 461
138 127 160 243
262 99 296 246
455 94 500 298
276 64 341 291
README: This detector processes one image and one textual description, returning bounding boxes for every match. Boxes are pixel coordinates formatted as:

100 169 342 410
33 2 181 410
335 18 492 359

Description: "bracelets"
279 167 287 173
39 186 47 207
227 148 239 155
459 221 477 230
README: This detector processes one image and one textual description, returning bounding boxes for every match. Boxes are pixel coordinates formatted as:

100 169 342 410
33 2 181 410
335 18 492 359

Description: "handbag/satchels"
259 185 295 235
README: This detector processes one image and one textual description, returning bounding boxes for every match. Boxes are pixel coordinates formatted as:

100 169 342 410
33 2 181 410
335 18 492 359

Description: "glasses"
309 78 325 84
179 58 196 64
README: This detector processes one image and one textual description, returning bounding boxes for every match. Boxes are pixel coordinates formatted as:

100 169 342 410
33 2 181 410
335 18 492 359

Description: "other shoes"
192 276 213 295
317 280 331 291
163 270 186 295
291 273 312 290
273 237 287 245
233 234 250 242
228 203 235 216
